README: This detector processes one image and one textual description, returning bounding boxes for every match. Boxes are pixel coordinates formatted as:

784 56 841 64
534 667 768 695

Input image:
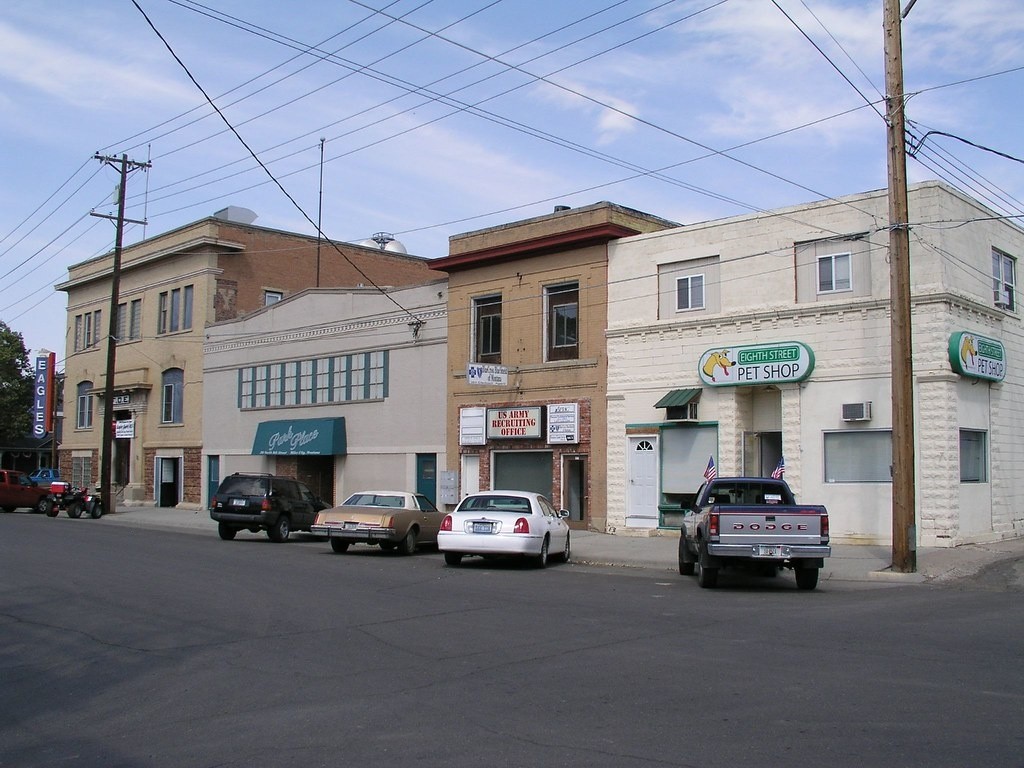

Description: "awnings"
250 417 347 456
653 388 702 409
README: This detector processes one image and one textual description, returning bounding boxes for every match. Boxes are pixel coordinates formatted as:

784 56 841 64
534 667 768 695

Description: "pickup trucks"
678 476 832 590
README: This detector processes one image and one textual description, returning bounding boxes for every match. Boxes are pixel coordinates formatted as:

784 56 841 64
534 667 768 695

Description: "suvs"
210 471 333 544
46 481 104 519
0 469 51 514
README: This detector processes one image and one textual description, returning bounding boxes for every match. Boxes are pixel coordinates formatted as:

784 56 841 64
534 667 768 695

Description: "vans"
20 467 60 485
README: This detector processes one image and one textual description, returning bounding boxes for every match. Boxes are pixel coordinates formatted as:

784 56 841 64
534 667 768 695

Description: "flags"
771 457 786 479
704 456 716 485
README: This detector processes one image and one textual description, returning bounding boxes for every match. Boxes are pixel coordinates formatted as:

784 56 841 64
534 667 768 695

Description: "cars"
436 489 572 570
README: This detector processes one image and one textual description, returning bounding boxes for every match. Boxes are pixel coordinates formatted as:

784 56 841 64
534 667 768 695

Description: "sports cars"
311 489 450 556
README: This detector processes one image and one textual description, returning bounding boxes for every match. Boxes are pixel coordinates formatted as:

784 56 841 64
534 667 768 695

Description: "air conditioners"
994 289 1010 306
841 401 871 421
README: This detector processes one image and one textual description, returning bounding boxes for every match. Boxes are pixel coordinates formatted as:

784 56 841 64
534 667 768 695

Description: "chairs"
714 494 730 504
